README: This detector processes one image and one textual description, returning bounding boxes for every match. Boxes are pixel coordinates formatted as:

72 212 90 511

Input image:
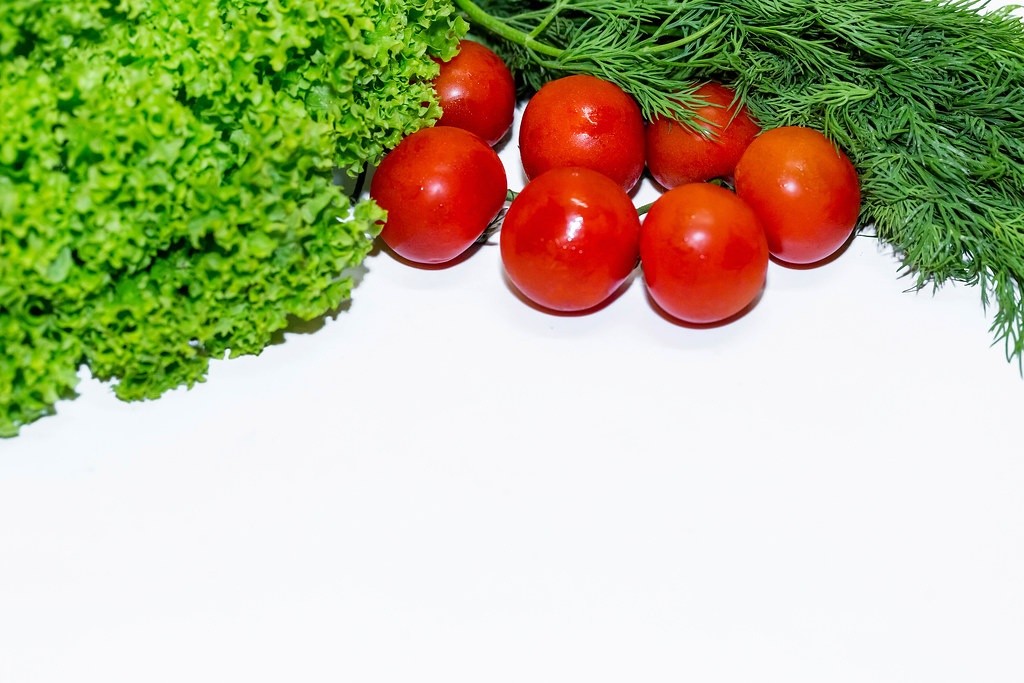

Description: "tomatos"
369 42 863 325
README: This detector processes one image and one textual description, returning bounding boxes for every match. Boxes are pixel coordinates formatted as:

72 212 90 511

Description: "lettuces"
1 0 469 445
